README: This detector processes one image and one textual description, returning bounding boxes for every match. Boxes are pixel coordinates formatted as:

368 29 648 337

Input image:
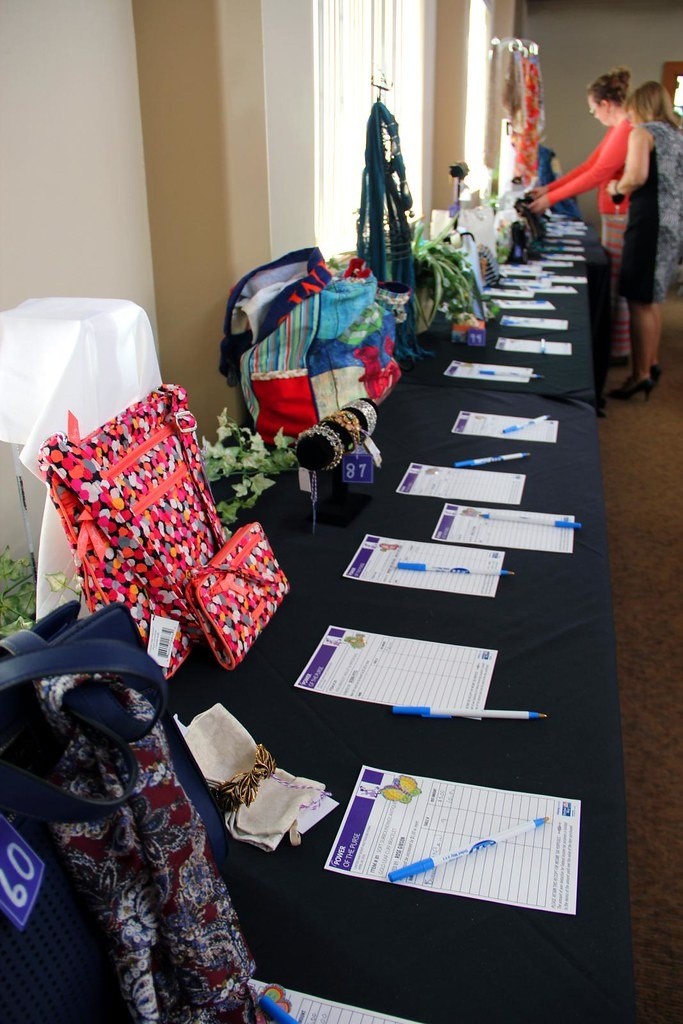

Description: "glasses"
589 103 599 116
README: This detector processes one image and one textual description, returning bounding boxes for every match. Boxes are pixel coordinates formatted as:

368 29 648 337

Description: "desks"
160 379 634 1023
401 215 597 400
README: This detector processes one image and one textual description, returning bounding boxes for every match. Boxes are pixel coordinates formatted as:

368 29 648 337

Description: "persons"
528 65 634 370
605 81 683 404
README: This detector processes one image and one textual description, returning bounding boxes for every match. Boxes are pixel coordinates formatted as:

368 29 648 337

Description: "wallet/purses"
185 522 291 669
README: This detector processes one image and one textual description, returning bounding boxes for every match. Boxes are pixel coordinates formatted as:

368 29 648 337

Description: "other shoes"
608 354 630 368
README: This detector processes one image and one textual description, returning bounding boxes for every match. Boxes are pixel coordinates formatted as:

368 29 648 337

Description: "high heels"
607 379 653 402
622 365 662 391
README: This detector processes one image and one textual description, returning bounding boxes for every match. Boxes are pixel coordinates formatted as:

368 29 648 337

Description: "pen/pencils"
453 452 531 472
259 994 301 1024
388 816 551 889
541 338 546 354
503 414 552 434
479 370 546 380
478 513 582 530
397 562 515 576
392 705 549 722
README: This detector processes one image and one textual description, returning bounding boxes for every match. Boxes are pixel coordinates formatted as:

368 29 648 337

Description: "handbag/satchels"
43 382 229 680
217 247 401 446
415 189 552 363
0 600 256 1024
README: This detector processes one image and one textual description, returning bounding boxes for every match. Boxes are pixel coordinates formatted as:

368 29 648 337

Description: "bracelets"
615 180 619 194
318 411 360 454
295 424 343 532
340 399 377 436
